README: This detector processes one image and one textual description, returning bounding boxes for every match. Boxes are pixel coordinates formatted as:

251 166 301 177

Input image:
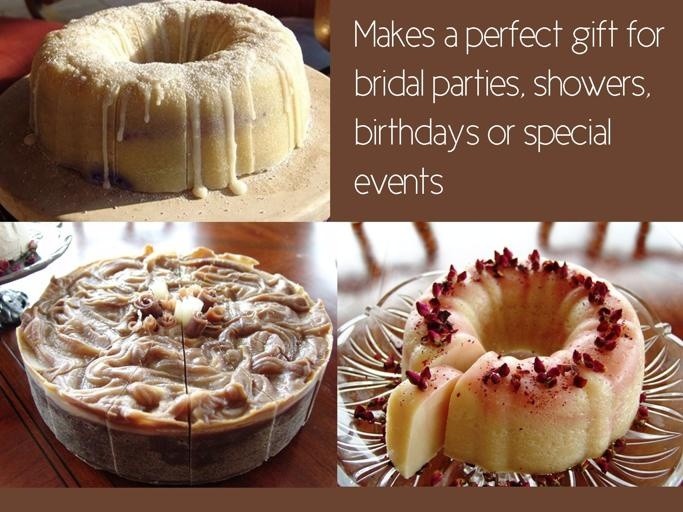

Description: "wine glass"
0 222 73 329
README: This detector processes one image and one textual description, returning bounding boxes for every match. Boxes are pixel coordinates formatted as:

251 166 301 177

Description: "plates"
336 263 683 489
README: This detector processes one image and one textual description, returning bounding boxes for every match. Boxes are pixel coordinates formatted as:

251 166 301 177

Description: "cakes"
14 242 337 485
24 0 308 198
384 247 646 479
